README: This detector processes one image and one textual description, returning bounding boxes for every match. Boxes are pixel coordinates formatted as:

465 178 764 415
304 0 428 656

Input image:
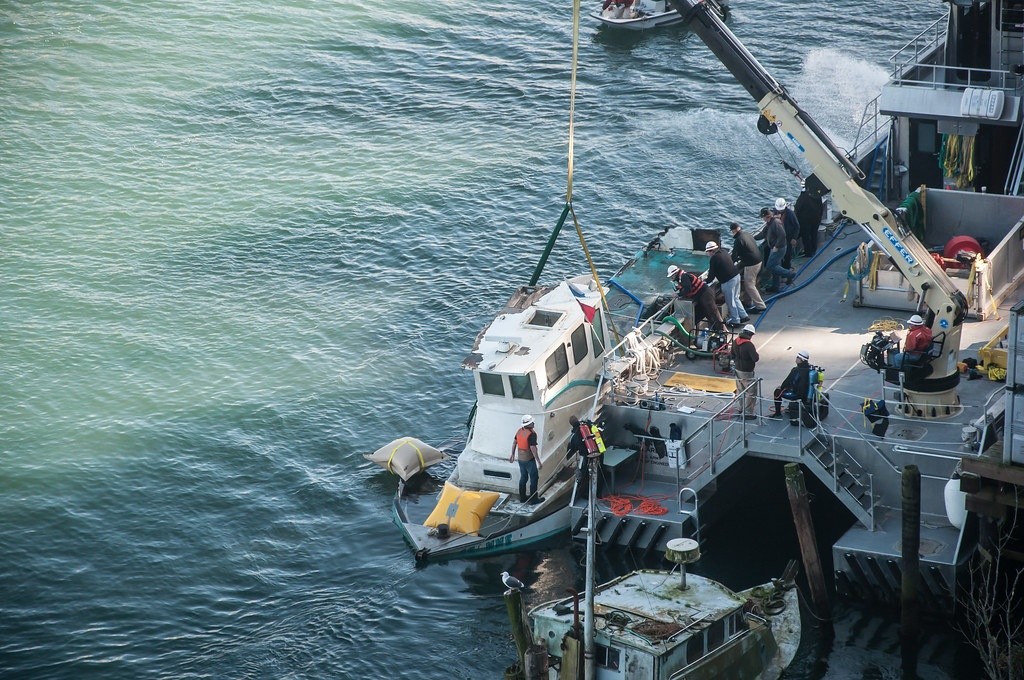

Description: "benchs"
789 392 829 428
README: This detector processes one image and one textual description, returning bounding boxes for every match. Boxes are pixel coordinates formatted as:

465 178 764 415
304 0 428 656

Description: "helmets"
667 265 680 278
775 198 786 210
521 415 535 426
743 324 755 333
797 350 809 360
907 315 924 326
706 241 719 252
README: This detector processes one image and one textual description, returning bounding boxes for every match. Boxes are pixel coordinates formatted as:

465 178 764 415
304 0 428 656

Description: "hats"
730 223 738 231
760 208 770 218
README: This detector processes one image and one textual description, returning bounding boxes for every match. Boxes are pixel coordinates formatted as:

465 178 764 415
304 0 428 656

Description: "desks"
603 445 638 495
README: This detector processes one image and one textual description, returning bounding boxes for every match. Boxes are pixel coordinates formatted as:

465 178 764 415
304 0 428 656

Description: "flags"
565 279 601 324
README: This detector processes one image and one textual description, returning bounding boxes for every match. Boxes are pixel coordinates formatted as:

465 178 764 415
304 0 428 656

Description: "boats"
589 0 730 30
392 206 729 567
569 0 1024 617
503 535 802 680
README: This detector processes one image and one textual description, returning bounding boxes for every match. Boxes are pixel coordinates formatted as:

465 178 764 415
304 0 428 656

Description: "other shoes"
729 323 740 328
766 286 779 292
749 307 766 314
520 495 530 503
786 271 795 284
531 497 545 504
745 415 756 420
740 317 750 324
769 412 781 418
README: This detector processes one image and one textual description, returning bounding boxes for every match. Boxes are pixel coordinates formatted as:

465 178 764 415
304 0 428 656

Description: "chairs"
886 331 946 370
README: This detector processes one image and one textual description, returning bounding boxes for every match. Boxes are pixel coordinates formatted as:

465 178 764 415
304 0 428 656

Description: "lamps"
589 278 598 292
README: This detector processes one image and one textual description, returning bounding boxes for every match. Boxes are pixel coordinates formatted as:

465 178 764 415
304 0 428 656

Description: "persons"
510 415 546 505
893 315 933 368
794 184 823 256
753 208 796 294
730 223 767 315
599 0 639 19
703 241 750 328
769 352 812 419
730 325 759 420
772 198 800 278
667 265 729 339
567 415 605 500
